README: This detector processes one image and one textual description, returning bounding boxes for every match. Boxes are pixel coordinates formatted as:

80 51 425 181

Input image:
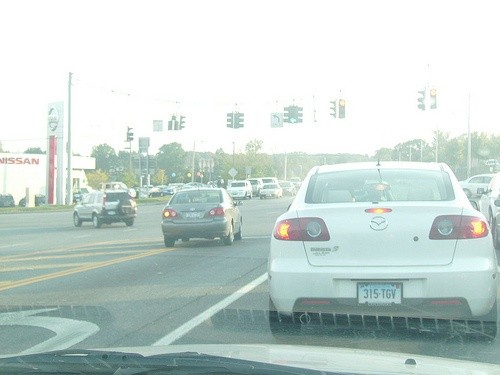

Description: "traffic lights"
126 126 134 141
226 112 233 129
429 89 436 110
339 99 346 118
418 89 425 111
180 115 185 129
330 100 337 119
292 106 304 123
282 105 291 123
234 112 245 129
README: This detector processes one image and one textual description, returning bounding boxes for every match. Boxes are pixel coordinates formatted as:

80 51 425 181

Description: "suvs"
72 189 139 227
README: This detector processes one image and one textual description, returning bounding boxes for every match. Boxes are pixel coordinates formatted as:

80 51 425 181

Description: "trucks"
0 153 96 208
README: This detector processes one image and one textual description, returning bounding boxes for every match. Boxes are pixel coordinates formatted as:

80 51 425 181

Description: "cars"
476 172 499 247
75 181 218 203
266 162 498 343
227 180 253 200
458 173 495 204
259 182 283 200
278 177 303 195
163 185 244 247
262 177 278 185
245 178 264 197
